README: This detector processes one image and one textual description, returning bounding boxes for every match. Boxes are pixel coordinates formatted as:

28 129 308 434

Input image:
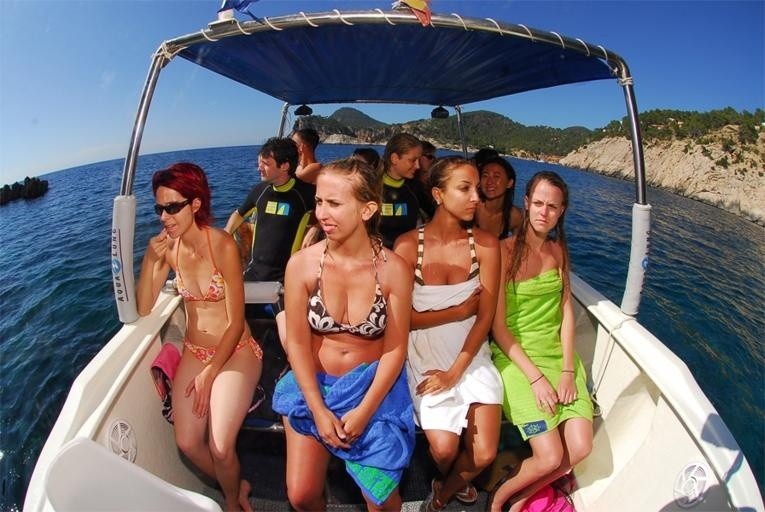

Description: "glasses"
422 152 433 161
153 199 194 216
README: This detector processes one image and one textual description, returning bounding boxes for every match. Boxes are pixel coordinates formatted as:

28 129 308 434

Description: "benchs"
171 319 598 433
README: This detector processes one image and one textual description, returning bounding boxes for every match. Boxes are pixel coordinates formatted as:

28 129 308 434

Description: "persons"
393 154 505 512
134 161 268 510
269 154 416 511
482 170 597 511
219 126 527 319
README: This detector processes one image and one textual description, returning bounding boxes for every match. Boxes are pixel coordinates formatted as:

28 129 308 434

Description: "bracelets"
530 373 545 385
562 367 576 375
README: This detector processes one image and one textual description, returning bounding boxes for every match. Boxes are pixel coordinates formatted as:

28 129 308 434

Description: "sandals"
417 474 480 512
